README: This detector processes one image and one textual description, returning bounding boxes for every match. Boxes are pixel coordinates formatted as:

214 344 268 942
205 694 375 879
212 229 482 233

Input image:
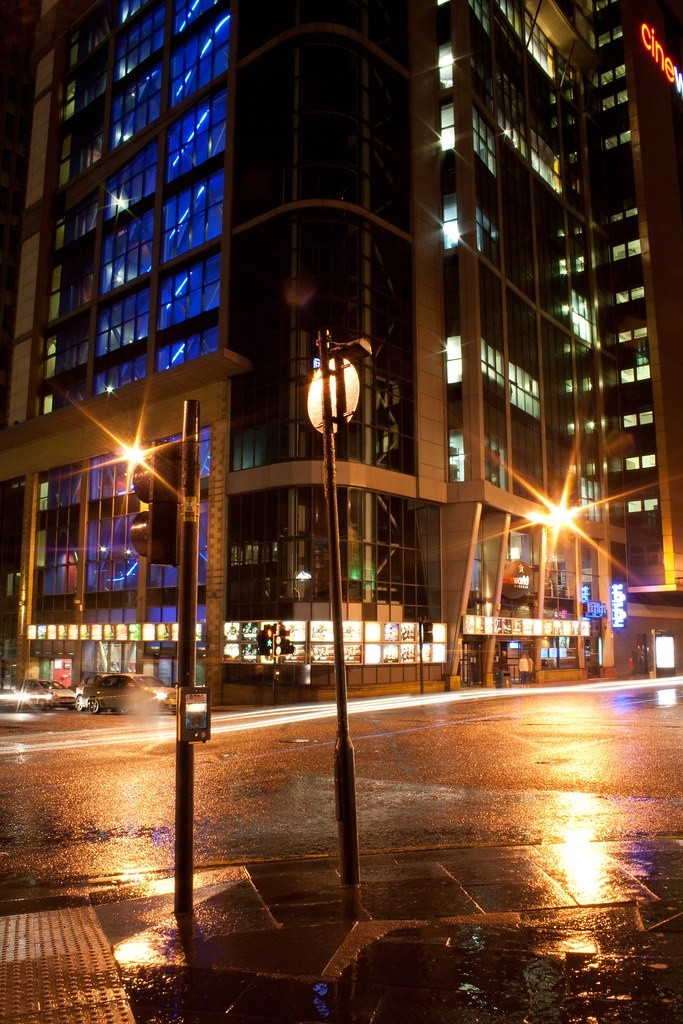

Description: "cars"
75 674 112 712
21 679 76 711
79 674 176 716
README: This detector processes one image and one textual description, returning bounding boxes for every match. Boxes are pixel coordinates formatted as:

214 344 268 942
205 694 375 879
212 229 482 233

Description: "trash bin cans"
502 671 512 688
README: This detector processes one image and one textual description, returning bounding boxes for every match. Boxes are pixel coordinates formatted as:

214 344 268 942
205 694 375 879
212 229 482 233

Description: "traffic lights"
275 634 295 655
264 624 273 657
